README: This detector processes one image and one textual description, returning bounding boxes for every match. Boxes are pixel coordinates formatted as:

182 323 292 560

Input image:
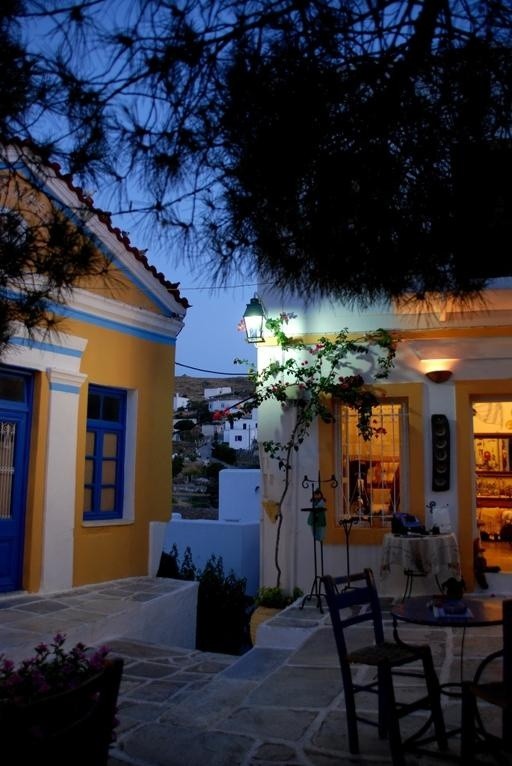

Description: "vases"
2 658 123 766
244 604 282 646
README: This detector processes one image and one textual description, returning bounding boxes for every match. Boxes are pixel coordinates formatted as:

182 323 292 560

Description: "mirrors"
474 431 512 476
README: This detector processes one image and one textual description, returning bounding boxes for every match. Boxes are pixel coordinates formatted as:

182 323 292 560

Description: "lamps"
425 369 451 383
242 293 270 343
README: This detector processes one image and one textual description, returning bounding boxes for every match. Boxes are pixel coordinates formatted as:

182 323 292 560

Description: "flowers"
209 305 394 602
1 634 111 694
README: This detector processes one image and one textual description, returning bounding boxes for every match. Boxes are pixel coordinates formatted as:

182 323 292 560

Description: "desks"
390 592 502 765
379 532 459 601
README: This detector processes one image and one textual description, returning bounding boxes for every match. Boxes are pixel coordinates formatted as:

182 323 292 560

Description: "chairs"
456 598 512 765
322 567 447 765
